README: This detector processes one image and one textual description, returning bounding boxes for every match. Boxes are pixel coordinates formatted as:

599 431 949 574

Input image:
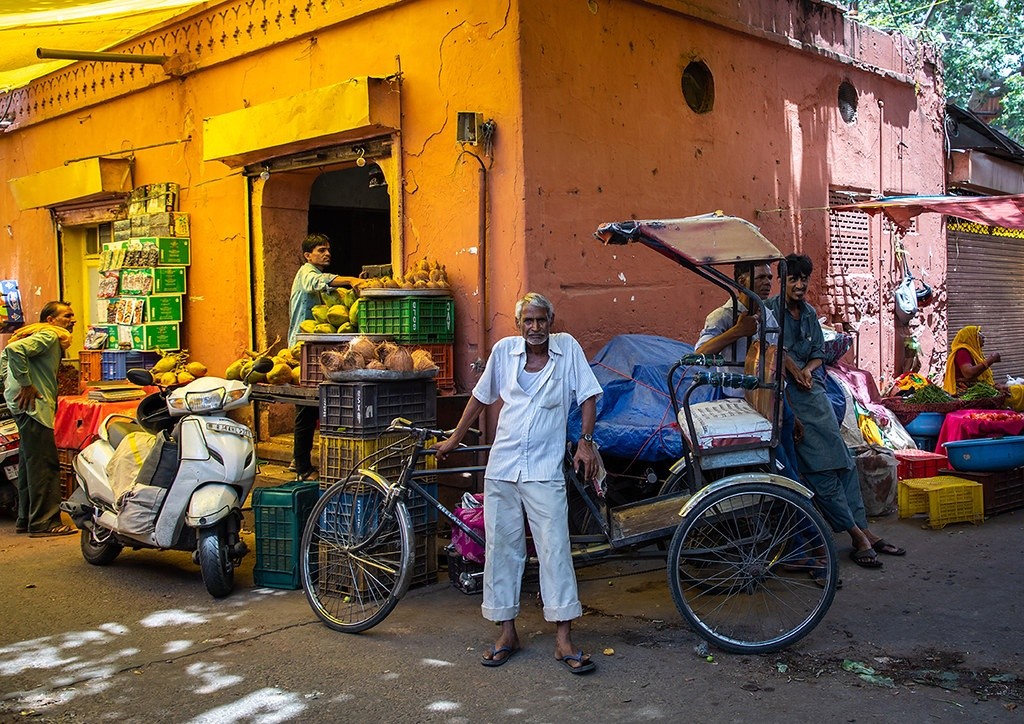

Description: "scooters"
61 357 276 600
1 374 22 522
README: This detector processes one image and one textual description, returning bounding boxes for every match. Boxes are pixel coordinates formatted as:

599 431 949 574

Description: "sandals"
15 526 30 534
28 525 79 536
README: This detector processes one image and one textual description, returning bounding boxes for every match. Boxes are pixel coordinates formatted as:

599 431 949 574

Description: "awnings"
760 192 1024 239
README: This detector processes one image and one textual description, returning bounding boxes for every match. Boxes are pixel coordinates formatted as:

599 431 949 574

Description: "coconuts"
151 257 451 385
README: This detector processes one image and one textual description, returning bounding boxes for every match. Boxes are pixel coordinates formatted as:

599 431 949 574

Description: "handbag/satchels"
903 252 932 303
894 244 918 325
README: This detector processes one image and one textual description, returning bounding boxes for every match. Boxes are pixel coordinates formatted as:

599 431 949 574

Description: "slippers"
558 649 596 674
810 568 843 589
871 538 907 556
849 549 884 568
781 557 824 572
480 646 521 667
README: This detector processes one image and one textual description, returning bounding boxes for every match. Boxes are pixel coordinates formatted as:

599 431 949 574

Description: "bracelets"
982 361 989 370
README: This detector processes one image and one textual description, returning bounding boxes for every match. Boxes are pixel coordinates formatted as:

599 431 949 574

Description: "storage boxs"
243 288 484 606
54 181 194 504
0 280 25 334
892 446 948 483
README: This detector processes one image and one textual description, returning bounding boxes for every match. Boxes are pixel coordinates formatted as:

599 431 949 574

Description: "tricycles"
299 212 842 655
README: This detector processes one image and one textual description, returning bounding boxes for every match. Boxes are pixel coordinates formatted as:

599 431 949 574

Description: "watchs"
578 433 593 442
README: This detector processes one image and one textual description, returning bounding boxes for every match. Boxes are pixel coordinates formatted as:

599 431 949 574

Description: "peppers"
904 383 1001 404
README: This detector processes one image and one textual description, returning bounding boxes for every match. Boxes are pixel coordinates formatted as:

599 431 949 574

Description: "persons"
1 301 79 539
945 325 1001 397
286 232 370 483
429 292 604 674
757 251 905 568
695 258 844 592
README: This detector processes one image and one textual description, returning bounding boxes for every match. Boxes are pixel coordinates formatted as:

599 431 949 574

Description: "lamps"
259 164 270 182
355 147 367 167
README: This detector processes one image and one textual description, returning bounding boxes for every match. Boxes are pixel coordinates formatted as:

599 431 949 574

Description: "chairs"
672 340 786 501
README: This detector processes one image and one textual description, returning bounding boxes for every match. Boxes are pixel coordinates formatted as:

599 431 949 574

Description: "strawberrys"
963 413 1024 421
